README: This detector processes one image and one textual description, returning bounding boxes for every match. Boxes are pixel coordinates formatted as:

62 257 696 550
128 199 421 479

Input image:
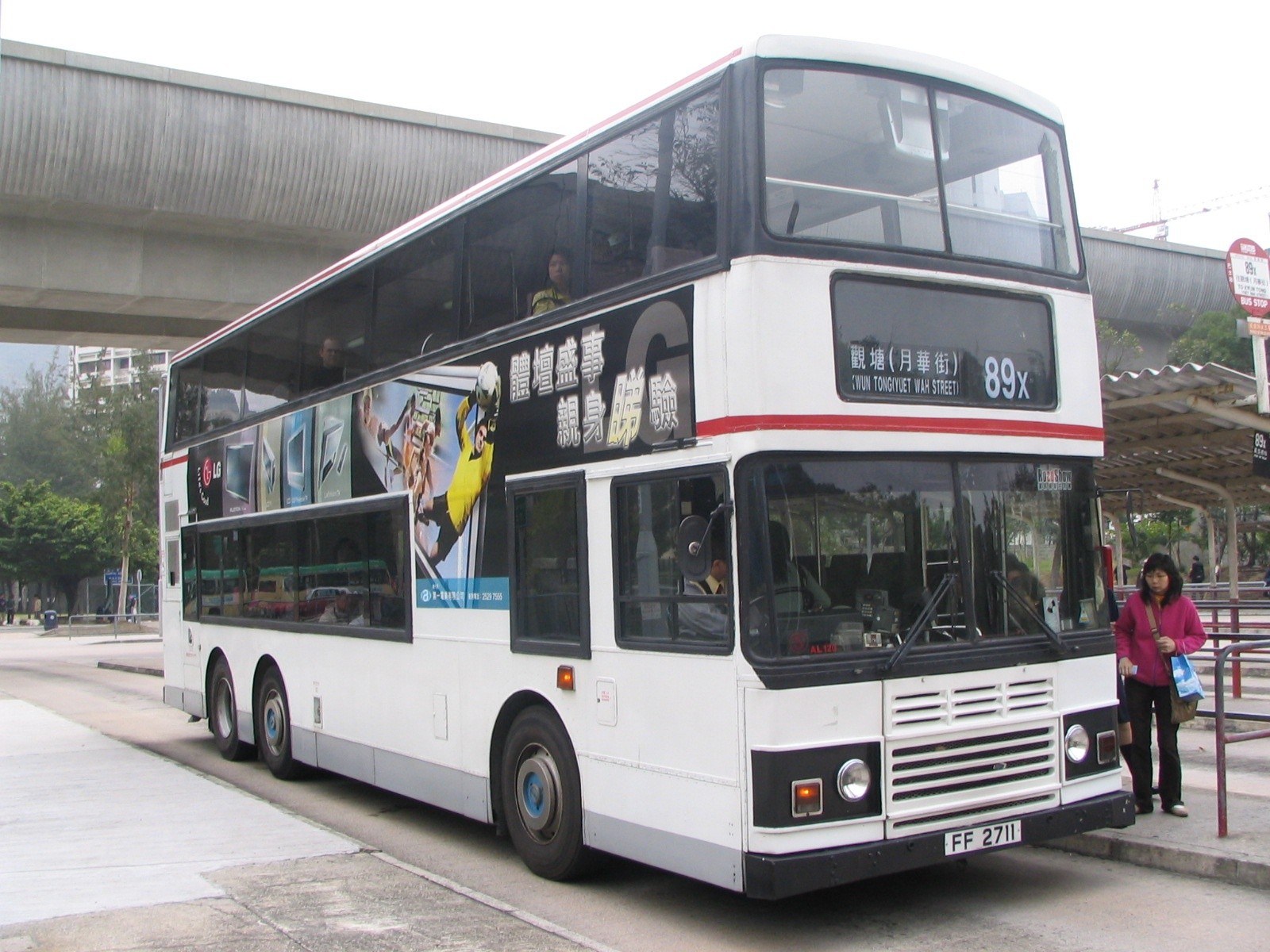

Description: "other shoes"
1171 804 1188 817
1135 807 1142 815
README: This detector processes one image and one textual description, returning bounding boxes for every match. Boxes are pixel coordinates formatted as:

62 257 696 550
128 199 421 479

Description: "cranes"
1115 179 1212 242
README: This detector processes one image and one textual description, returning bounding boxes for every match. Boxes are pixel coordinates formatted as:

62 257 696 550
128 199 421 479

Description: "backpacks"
1193 567 1204 582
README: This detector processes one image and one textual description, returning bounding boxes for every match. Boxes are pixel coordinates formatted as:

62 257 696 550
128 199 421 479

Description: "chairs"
793 550 960 606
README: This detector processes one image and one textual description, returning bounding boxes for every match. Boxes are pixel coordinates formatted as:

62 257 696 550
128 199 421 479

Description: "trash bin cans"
44 610 58 631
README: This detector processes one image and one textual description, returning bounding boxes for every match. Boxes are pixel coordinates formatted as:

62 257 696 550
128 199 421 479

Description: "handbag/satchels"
1169 673 1199 724
1171 652 1205 701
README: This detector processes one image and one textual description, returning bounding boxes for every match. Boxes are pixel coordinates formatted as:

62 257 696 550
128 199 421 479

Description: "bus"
155 35 1136 908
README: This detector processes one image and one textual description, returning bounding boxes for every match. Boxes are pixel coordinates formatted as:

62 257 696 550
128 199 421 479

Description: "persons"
354 386 498 563
676 519 835 641
1004 553 1207 817
532 244 582 317
298 335 351 393
229 564 373 630
